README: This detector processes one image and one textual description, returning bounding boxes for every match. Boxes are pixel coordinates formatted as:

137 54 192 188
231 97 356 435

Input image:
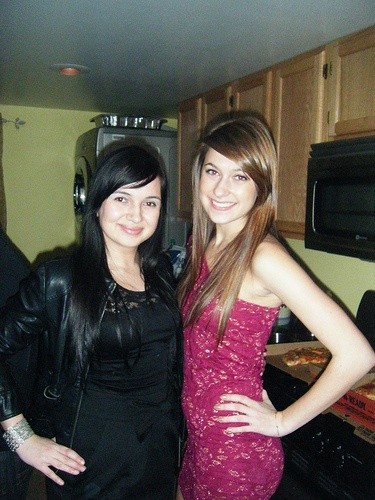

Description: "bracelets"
274 410 280 437
3 417 35 453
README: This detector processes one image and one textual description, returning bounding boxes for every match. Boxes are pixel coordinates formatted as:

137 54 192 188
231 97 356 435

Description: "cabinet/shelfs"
326 23 375 142
176 83 231 221
232 43 326 240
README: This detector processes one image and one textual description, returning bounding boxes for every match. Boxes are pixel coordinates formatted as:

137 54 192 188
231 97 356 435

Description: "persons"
177 115 375 500
1 144 185 500
0 228 57 500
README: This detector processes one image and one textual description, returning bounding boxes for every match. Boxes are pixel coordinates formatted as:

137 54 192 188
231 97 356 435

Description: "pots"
91 113 169 130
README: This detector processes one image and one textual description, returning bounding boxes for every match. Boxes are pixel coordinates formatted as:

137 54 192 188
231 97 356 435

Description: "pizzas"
282 347 332 366
354 378 375 401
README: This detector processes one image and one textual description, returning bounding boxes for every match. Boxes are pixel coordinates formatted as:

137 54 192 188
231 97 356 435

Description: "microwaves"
305 136 375 262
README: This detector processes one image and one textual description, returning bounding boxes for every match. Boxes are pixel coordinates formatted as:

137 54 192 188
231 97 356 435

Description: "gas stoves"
261 290 375 500
